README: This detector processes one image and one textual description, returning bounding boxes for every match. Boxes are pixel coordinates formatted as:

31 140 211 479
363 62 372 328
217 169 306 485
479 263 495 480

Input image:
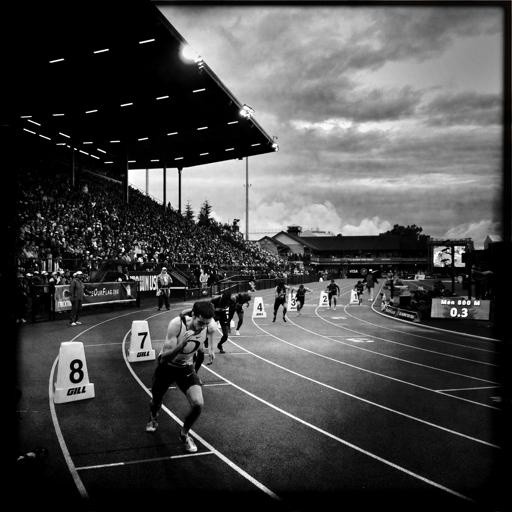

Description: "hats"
73 271 82 276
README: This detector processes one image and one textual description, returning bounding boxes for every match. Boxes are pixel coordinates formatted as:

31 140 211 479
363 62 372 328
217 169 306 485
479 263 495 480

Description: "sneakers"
145 413 159 432
283 317 286 322
217 344 224 353
71 321 81 326
272 318 276 322
179 430 198 453
235 329 239 335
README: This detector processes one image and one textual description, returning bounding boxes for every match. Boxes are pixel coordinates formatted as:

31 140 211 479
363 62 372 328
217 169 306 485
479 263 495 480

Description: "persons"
201 289 236 354
15 156 302 328
302 253 474 313
144 300 217 454
